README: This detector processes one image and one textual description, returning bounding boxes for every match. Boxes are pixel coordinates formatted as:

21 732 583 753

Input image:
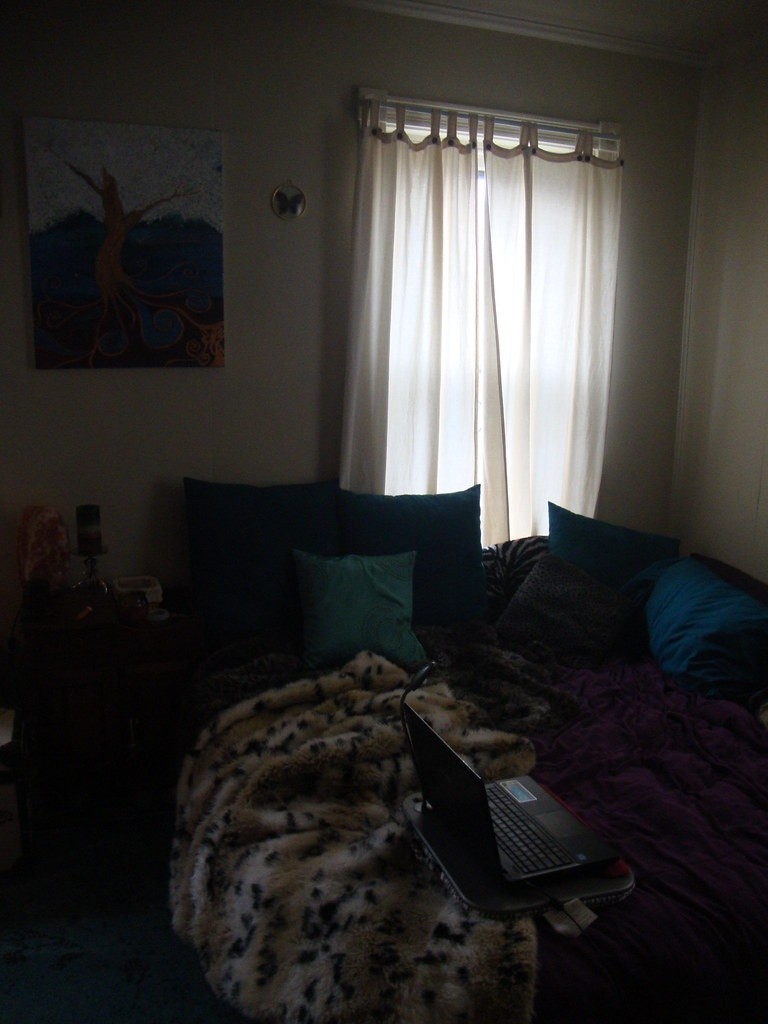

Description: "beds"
168 551 767 1024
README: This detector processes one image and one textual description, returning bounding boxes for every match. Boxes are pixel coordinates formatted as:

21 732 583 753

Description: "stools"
0 701 34 877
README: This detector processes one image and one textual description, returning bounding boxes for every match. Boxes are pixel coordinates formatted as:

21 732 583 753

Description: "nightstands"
26 607 203 825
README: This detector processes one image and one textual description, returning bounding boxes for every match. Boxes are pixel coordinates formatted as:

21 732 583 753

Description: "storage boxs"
113 575 163 610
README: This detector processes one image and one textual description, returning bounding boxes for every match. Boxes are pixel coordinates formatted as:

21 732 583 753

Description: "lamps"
76 504 108 596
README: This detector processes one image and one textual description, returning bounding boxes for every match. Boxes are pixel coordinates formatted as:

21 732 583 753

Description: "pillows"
185 477 340 648
548 502 682 662
495 560 640 670
291 548 428 672
339 486 490 628
643 558 768 700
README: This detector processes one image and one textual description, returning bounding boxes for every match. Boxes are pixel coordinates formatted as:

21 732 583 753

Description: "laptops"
404 702 619 893
